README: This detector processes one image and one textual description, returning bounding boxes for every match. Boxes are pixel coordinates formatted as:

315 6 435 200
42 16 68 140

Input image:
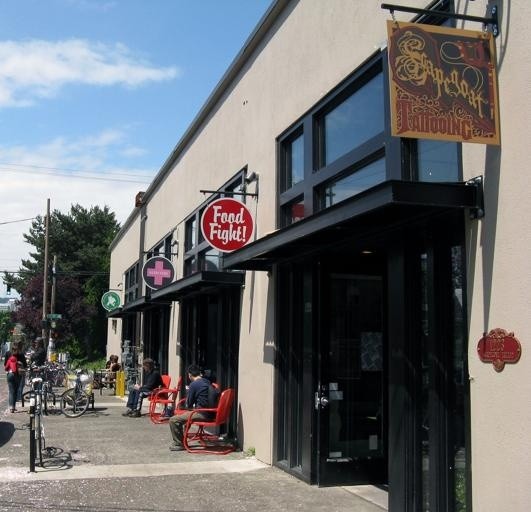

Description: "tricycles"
59 370 94 418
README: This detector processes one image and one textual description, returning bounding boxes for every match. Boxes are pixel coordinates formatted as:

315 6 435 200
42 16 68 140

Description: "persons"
168 364 217 451
122 357 163 417
102 355 120 388
2 336 46 413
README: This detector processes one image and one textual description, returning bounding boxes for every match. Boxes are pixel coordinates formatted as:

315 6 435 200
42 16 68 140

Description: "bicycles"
22 379 54 467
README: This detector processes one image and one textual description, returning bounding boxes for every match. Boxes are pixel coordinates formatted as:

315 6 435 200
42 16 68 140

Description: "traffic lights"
6 285 10 295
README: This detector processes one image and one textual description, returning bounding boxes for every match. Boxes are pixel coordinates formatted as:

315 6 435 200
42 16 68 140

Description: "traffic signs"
46 314 61 319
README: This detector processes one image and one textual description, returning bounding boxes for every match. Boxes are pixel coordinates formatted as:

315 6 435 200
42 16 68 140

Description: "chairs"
135 375 172 418
149 375 183 424
100 370 120 396
173 382 221 440
183 387 237 455
